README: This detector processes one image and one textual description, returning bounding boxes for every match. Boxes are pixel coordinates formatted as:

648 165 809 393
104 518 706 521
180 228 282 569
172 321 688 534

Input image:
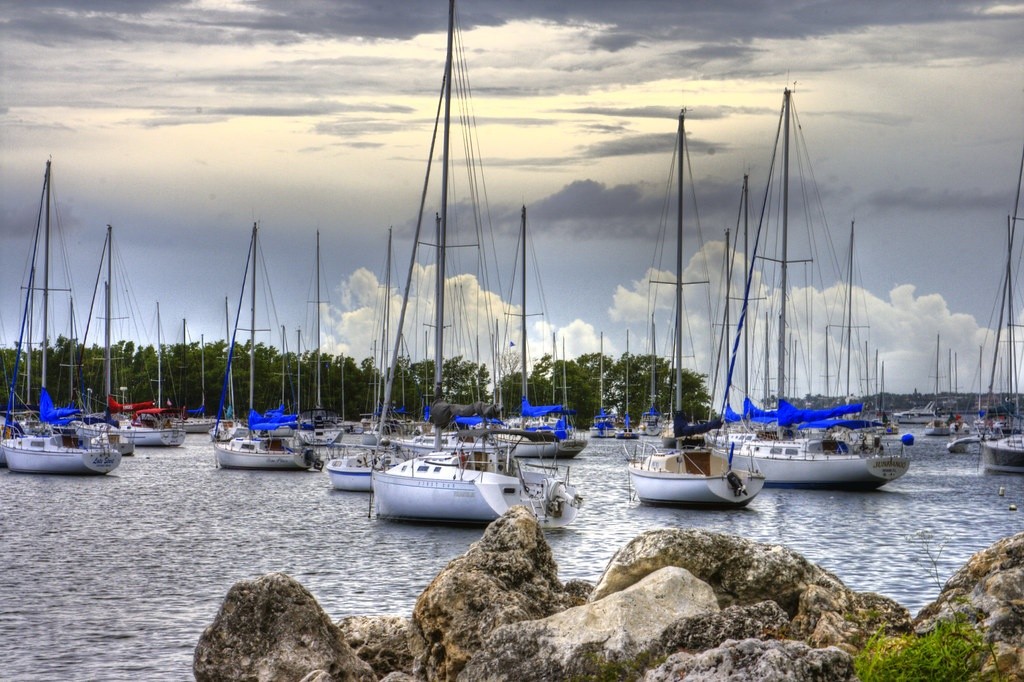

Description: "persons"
882 412 888 423
131 413 176 429
786 424 885 458
945 412 964 432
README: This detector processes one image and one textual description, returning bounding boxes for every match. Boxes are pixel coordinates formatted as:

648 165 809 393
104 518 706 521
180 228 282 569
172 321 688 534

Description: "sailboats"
0 1 1024 535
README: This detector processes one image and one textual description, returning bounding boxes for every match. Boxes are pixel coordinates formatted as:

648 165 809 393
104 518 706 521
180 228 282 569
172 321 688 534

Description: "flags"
167 399 172 407
511 341 515 347
